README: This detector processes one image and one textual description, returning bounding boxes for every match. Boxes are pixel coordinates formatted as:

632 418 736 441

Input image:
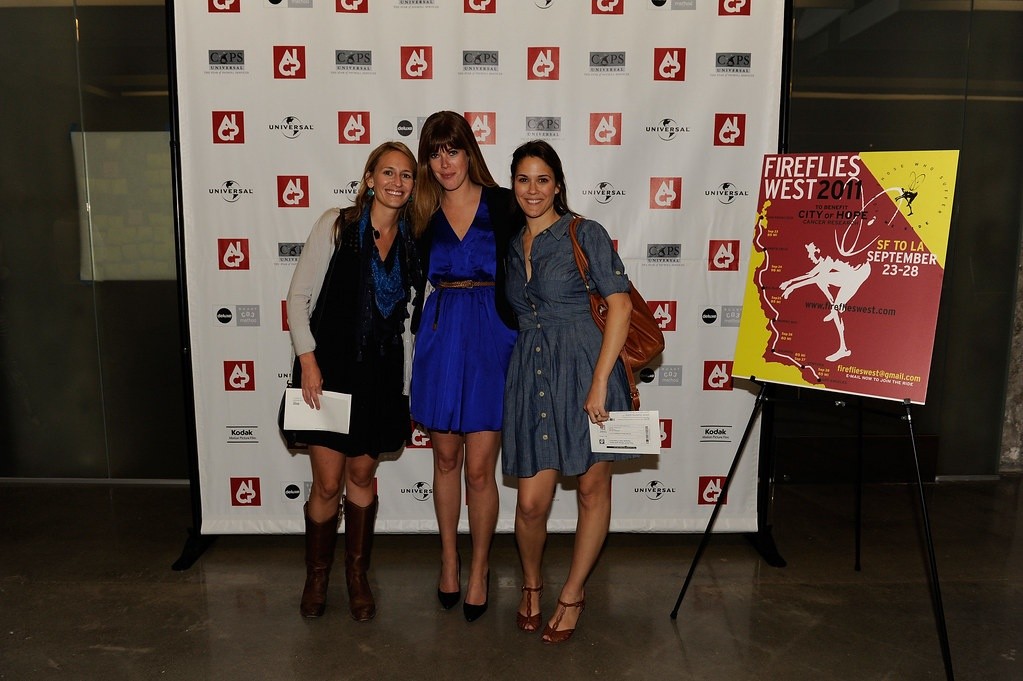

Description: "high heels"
517 579 544 634
438 551 461 610
463 568 490 622
541 590 586 645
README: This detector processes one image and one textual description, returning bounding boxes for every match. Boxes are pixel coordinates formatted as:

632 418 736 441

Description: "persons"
278 142 418 623
405 111 526 621
502 140 641 645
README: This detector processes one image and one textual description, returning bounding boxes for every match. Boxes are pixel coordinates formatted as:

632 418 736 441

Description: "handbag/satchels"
569 218 665 411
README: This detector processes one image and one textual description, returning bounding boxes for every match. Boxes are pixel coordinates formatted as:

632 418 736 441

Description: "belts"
435 280 496 288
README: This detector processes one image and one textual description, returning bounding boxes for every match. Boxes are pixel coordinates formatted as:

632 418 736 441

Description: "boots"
342 495 378 623
300 501 343 618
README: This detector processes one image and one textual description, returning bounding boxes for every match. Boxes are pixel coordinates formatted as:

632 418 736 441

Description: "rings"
595 413 600 418
